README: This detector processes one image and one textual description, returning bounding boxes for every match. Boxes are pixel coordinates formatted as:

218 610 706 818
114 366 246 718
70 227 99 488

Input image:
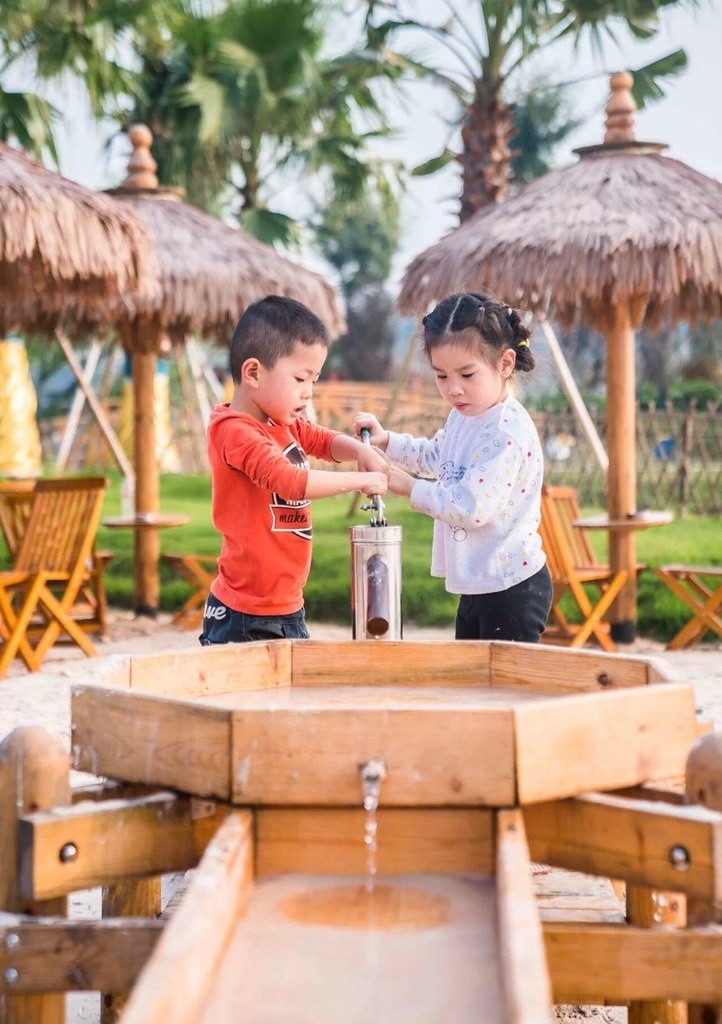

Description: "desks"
570 510 675 648
102 514 189 619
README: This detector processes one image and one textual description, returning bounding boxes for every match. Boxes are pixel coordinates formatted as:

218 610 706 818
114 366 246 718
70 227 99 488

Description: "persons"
351 287 556 644
196 295 391 644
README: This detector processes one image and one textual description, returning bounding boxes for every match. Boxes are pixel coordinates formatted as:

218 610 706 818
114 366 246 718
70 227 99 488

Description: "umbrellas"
88 121 351 620
1 141 145 344
396 68 722 646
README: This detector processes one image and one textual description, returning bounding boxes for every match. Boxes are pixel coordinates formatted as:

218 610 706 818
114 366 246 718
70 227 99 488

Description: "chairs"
0 476 115 680
161 553 218 632
538 483 629 653
656 563 722 651
549 487 646 620
